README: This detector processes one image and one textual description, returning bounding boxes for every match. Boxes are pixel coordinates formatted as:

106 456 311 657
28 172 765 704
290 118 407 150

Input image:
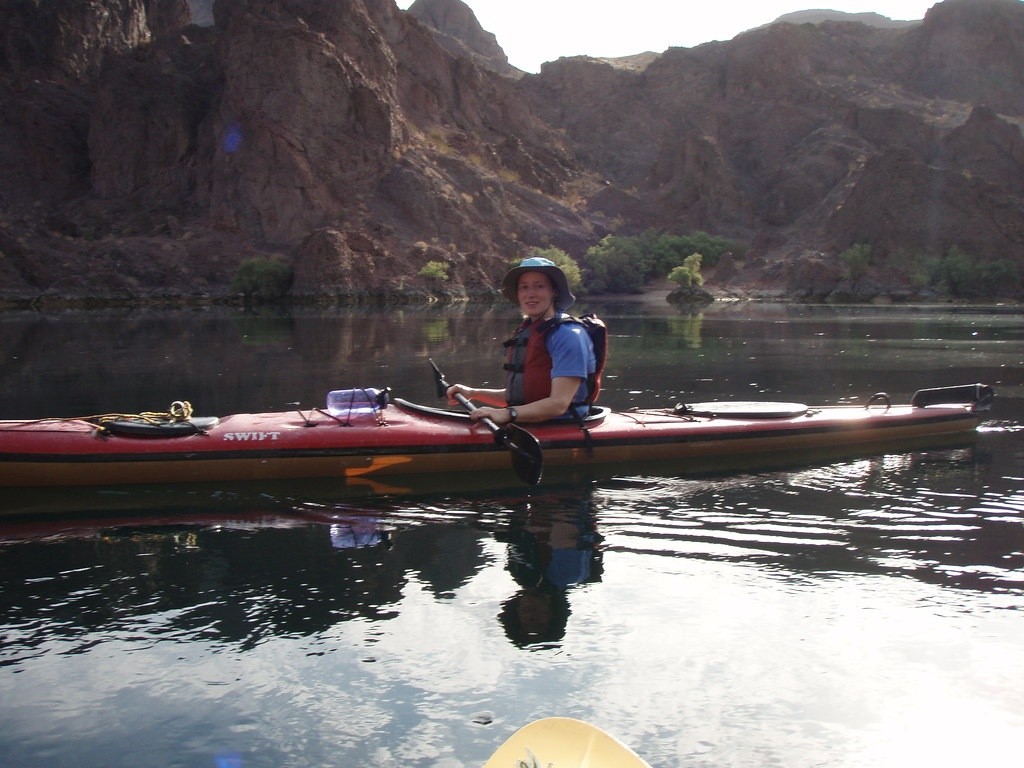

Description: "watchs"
505 407 518 423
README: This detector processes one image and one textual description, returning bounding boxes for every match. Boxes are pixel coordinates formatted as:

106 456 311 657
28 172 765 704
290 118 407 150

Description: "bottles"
327 388 391 416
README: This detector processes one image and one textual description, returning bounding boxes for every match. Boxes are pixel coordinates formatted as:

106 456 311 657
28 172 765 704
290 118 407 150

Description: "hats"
502 257 576 311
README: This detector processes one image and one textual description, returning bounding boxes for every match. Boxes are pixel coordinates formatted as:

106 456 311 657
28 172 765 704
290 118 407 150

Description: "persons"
447 256 596 424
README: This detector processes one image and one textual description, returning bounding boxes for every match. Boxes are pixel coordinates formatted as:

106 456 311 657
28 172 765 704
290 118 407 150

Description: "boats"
0 382 993 499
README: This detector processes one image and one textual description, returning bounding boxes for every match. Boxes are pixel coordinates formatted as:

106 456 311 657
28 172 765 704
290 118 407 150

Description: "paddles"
426 357 544 488
